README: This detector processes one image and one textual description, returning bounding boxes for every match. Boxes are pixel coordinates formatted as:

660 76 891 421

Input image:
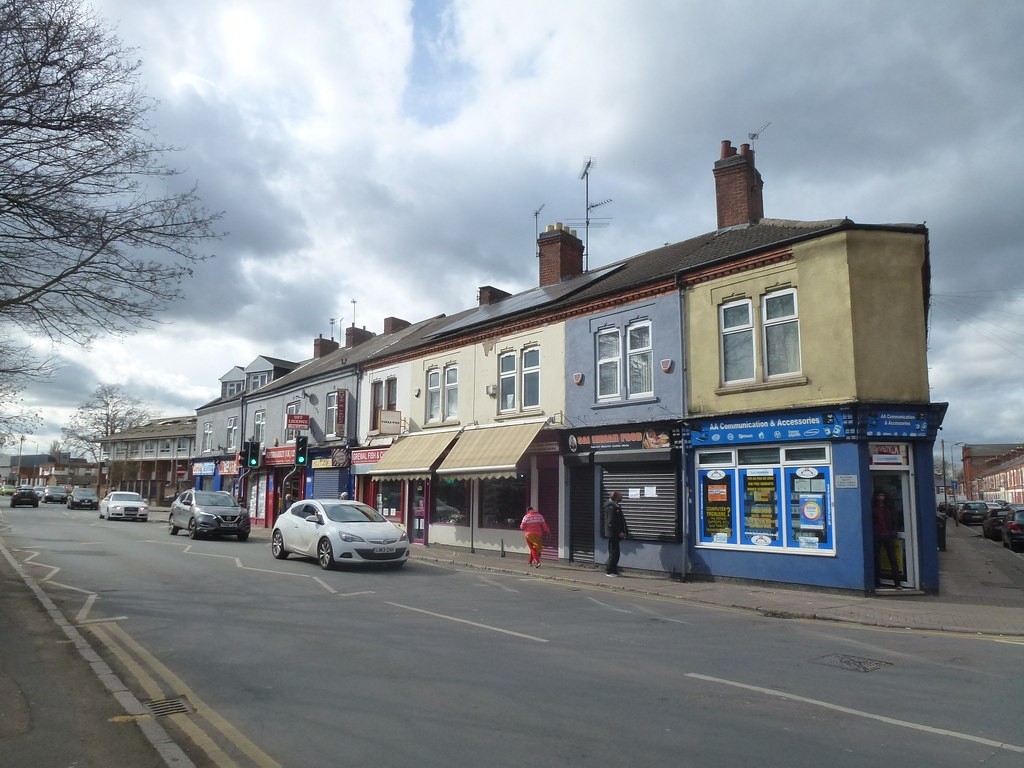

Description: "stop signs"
177 467 185 477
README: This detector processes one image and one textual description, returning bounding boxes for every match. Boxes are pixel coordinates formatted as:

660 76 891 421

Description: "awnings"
362 431 459 481
436 422 546 483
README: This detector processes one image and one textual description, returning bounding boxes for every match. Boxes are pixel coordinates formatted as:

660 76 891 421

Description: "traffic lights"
247 441 260 469
295 435 308 467
238 443 248 467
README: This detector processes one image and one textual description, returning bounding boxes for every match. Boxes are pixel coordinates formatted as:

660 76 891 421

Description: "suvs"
169 488 251 541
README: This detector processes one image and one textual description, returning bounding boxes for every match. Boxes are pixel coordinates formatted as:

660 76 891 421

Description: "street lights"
950 441 966 528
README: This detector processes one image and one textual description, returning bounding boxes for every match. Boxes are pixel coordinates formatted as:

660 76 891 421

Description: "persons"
281 494 297 513
867 491 901 589
340 492 348 500
519 506 551 568
104 486 115 497
604 492 629 578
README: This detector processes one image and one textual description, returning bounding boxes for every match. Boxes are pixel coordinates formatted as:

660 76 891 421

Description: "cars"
270 499 412 571
10 487 39 508
98 491 150 522
938 497 1024 553
66 487 99 510
20 484 68 504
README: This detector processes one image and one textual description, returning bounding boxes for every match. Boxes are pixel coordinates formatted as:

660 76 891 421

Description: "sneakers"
605 572 622 578
534 563 541 568
525 564 532 566
